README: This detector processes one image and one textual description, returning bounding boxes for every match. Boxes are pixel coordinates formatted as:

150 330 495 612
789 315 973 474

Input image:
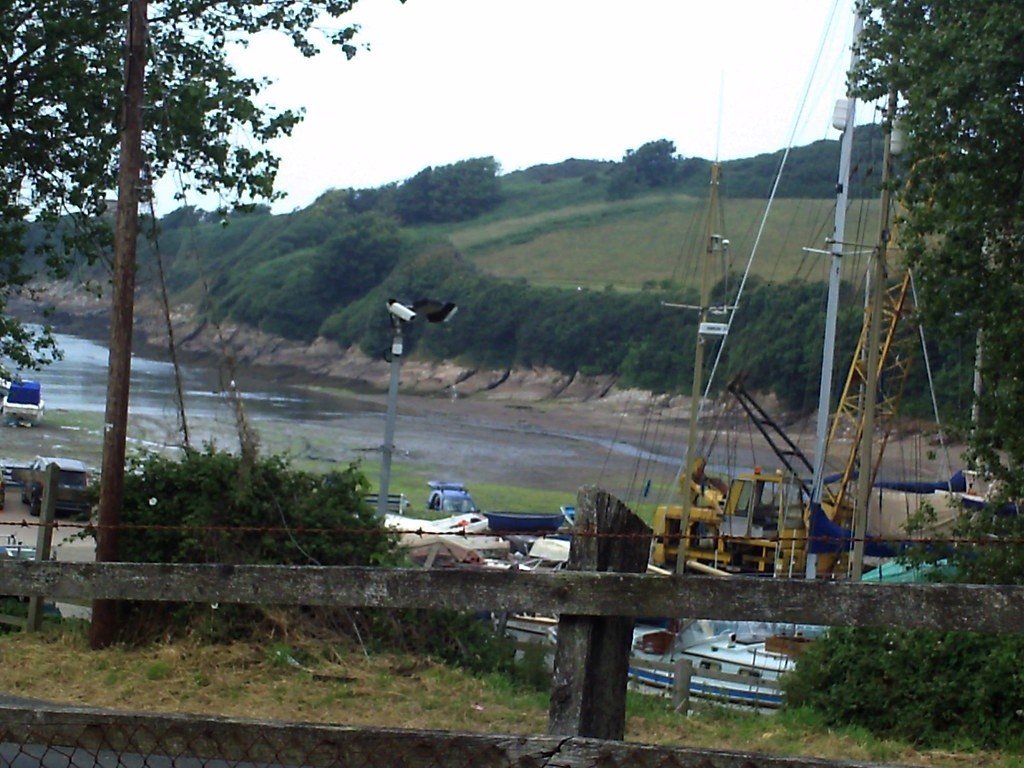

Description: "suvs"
427 480 481 513
21 455 94 521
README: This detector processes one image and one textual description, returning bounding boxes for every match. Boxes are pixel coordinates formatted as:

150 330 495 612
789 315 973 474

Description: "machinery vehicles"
646 154 964 580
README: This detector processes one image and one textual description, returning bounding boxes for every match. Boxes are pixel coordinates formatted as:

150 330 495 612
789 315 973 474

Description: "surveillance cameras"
386 299 417 322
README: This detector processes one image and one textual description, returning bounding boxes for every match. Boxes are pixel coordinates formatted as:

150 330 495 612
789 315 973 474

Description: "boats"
493 612 825 711
483 511 566 533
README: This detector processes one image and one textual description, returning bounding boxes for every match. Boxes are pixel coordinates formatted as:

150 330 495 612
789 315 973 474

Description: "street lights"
375 294 459 527
